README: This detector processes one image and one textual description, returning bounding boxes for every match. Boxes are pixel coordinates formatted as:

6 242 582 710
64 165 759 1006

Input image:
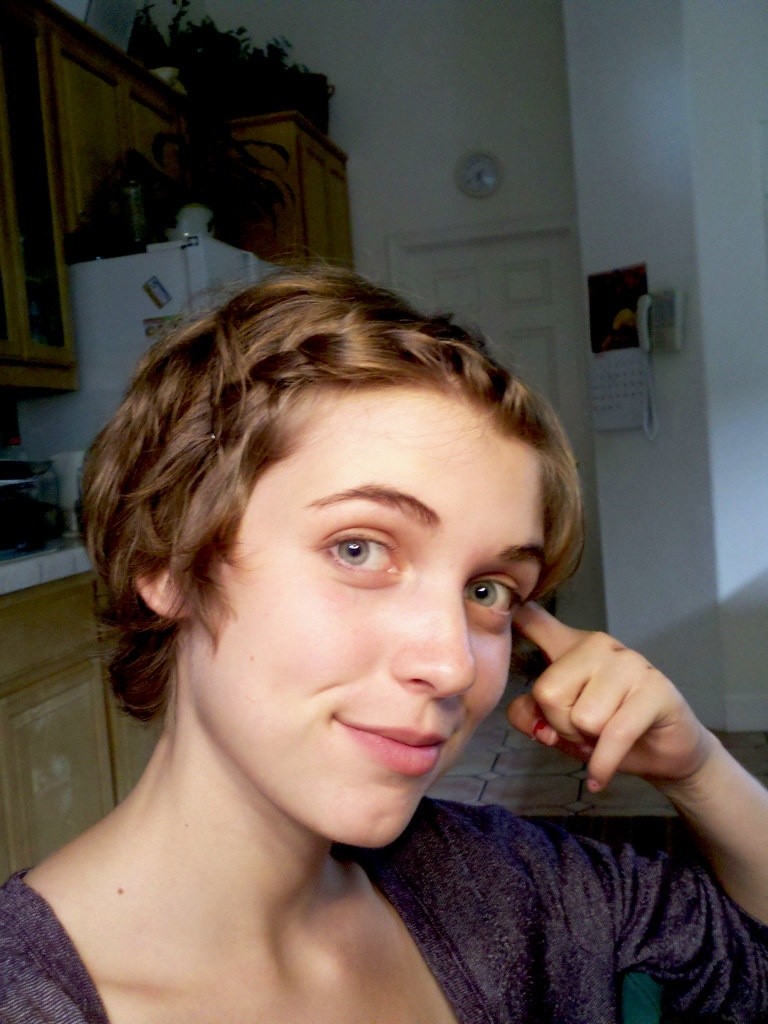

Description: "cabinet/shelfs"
1 502 348 914
0 0 354 386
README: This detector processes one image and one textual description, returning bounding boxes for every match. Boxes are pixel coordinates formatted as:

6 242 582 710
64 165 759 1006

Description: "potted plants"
59 137 298 238
117 1 314 122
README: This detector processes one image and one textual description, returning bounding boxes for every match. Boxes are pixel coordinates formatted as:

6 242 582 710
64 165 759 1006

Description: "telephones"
636 287 685 352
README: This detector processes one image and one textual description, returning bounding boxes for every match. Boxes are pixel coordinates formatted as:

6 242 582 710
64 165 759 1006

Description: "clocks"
455 150 506 200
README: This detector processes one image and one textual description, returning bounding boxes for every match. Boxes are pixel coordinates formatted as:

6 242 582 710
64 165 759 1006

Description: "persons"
0 271 767 1024
